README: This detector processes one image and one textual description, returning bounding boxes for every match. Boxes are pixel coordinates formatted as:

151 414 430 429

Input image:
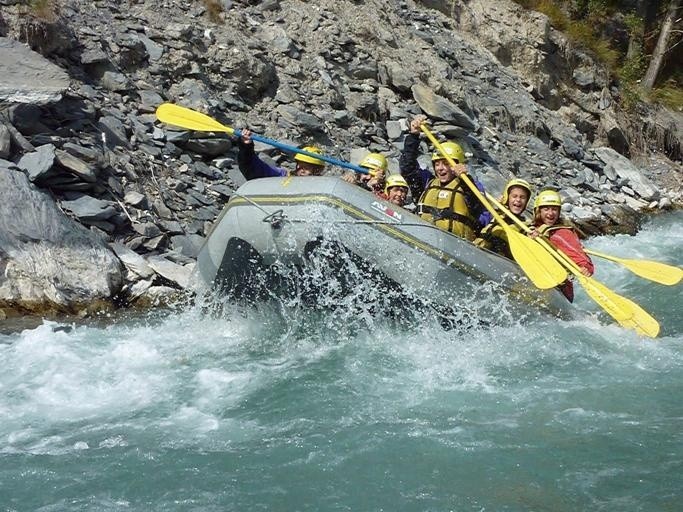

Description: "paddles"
484 191 661 340
420 123 566 289
156 104 379 178
583 248 683 286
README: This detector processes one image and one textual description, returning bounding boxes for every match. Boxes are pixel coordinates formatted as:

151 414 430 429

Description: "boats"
192 176 608 334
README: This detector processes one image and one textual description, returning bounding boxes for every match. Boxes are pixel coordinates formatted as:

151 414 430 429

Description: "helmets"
384 173 410 197
294 146 326 169
431 141 465 166
533 189 563 210
502 178 532 212
359 153 388 174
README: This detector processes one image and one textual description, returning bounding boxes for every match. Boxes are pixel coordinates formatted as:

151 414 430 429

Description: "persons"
398 114 488 243
380 174 409 207
232 129 325 181
532 190 594 304
343 153 388 194
472 175 539 262
